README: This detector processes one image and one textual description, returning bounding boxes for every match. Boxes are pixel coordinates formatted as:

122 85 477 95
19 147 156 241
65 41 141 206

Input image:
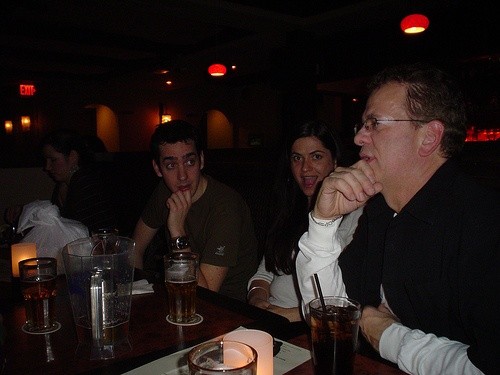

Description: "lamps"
400 14 430 33
207 64 226 76
223 329 274 375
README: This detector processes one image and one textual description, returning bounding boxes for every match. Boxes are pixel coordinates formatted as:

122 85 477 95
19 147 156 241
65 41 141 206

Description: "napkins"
117 279 154 295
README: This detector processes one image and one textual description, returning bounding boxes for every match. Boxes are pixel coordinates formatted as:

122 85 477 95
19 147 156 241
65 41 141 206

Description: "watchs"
171 237 188 251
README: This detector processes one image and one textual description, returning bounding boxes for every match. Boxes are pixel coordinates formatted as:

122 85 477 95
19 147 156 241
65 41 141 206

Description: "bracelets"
247 287 269 301
311 211 342 226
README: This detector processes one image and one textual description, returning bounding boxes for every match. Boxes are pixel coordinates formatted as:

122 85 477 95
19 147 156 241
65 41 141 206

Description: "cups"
17 256 58 331
309 295 361 375
61 234 136 361
163 252 199 324
186 340 258 375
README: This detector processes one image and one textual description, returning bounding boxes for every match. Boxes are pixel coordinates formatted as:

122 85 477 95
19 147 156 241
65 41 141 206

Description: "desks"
0 265 411 375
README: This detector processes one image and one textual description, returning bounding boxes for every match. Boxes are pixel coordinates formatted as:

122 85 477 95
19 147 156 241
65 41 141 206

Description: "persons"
6 128 119 238
295 65 500 375
247 122 367 322
127 119 260 302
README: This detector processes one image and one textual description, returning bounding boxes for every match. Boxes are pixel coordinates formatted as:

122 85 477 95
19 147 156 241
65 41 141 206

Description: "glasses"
354 118 435 136
